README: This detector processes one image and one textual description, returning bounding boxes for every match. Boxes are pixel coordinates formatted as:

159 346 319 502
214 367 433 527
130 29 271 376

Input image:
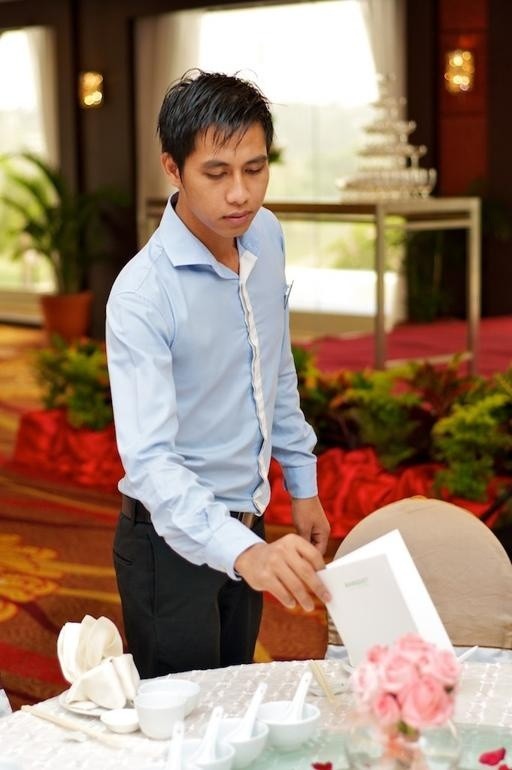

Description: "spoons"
223 682 268 740
189 706 224 763
280 671 313 723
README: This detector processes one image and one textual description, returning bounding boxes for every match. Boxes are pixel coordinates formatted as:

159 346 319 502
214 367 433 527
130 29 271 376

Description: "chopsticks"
20 705 121 749
312 661 336 704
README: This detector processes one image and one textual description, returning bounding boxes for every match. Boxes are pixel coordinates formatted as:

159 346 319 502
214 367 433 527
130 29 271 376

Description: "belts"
120 494 263 530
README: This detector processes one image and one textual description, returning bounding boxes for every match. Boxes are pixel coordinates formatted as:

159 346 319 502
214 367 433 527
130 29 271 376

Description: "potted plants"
2 145 138 350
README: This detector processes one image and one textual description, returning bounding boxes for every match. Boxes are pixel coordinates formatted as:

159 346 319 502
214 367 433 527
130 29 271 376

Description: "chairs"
317 493 512 651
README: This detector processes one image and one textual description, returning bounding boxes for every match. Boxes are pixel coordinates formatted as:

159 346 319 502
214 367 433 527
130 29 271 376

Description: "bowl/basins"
201 718 269 769
173 738 234 769
138 678 200 717
100 709 138 734
258 701 320 752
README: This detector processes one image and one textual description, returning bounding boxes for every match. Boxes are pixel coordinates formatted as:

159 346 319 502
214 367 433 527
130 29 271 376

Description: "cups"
135 692 187 741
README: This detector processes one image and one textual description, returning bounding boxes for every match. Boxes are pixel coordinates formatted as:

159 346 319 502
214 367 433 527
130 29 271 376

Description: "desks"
147 193 484 372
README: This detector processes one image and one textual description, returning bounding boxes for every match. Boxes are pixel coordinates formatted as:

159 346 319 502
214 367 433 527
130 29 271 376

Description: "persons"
104 65 334 680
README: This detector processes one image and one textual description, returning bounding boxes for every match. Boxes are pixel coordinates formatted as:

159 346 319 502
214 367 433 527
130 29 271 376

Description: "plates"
59 686 136 715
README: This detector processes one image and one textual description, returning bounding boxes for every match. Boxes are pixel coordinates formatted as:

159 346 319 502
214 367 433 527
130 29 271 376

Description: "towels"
54 610 139 713
